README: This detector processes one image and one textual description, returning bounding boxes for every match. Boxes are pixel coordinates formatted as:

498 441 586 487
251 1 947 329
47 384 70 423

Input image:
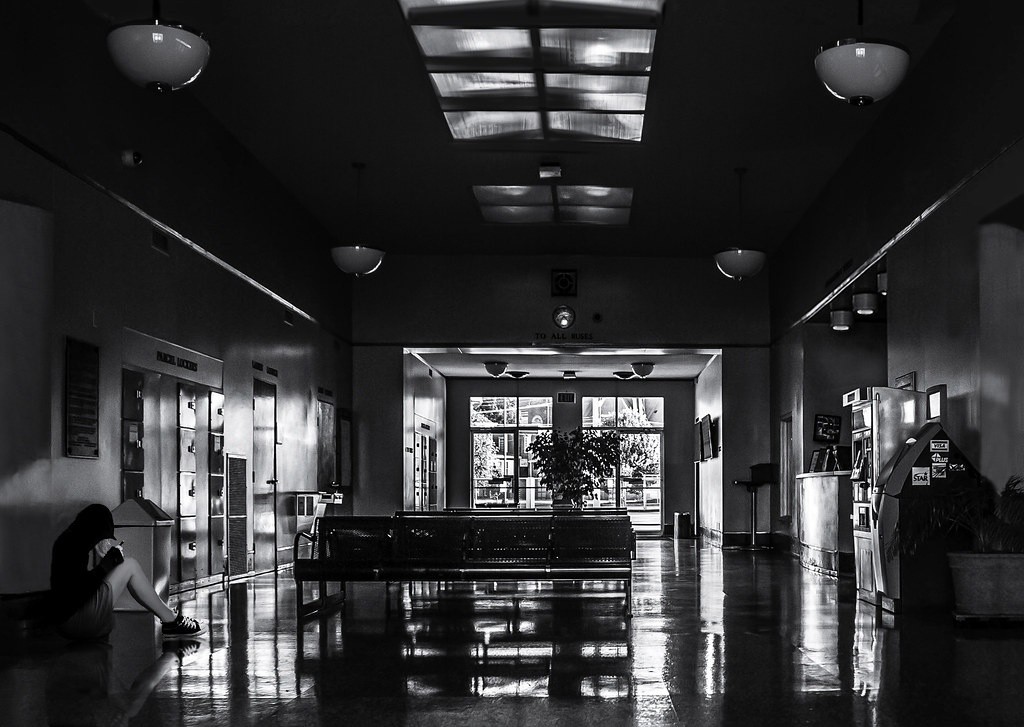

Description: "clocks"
552 304 576 329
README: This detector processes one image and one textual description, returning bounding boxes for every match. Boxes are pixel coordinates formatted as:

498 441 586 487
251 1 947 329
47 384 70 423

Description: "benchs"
293 506 637 634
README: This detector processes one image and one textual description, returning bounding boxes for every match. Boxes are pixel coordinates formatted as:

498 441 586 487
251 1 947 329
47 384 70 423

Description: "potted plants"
886 464 1024 616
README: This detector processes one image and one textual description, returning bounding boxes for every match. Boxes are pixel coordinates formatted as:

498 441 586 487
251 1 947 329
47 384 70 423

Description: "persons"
51 504 208 637
55 637 204 727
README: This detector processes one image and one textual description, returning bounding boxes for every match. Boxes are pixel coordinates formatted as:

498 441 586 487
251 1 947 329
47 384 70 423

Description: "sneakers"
162 636 209 662
161 609 210 641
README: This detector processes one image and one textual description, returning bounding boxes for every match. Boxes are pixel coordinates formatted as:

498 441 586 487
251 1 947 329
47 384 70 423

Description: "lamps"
105 0 215 97
539 161 561 178
614 372 633 378
813 1 912 109
714 166 768 283
851 281 877 315
506 372 529 377
631 362 655 377
330 162 387 278
484 361 509 376
562 370 576 379
878 262 888 296
830 299 855 331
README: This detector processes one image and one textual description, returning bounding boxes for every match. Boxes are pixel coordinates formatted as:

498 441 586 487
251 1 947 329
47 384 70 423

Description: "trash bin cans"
674 511 691 539
108 498 175 611
